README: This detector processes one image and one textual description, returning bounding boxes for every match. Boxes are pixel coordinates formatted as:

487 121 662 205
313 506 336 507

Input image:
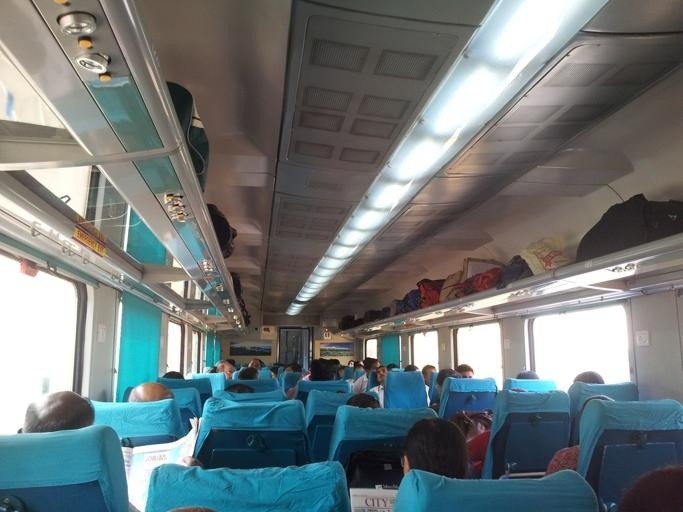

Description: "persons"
15 390 98 432
126 356 614 486
616 466 683 509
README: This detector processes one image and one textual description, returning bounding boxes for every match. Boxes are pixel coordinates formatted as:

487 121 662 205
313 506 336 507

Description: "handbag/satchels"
454 410 493 433
167 81 210 192
208 204 238 258
